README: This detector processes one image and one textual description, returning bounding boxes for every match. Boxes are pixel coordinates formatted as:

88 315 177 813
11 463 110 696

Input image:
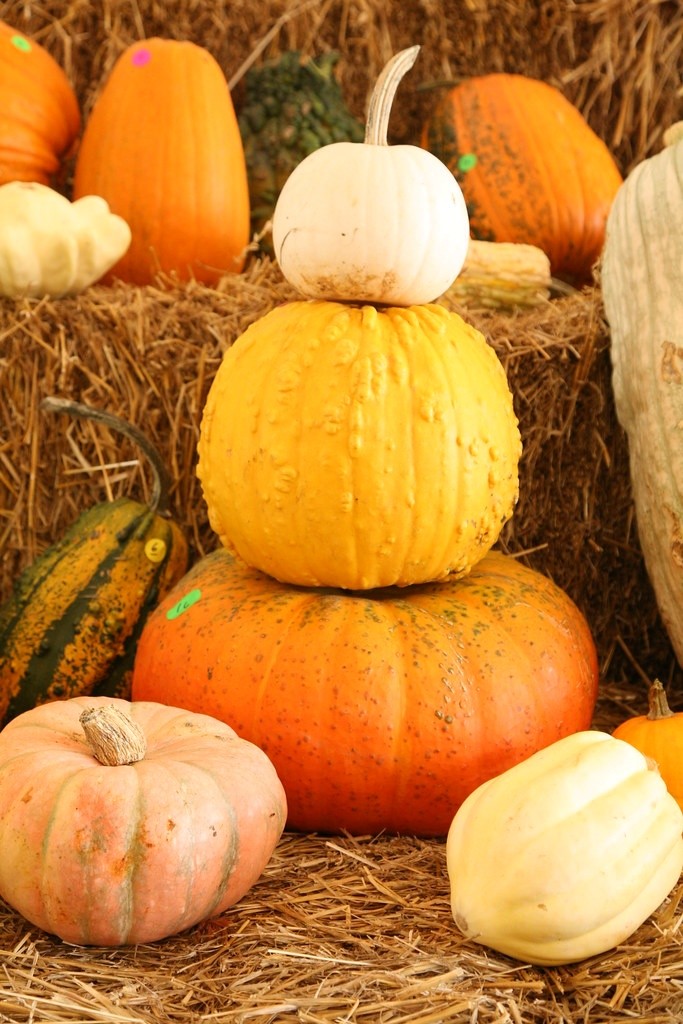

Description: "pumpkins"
1 15 682 969
